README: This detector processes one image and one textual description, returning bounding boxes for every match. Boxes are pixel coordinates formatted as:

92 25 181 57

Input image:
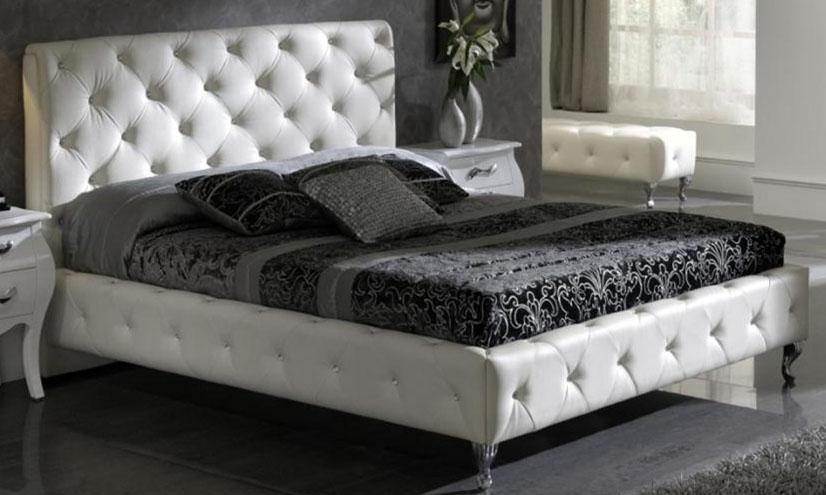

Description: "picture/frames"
428 2 518 64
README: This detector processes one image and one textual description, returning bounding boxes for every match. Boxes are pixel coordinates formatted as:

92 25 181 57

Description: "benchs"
543 102 698 209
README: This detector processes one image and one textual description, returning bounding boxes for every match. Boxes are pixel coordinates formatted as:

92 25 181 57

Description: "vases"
436 89 468 147
452 73 485 145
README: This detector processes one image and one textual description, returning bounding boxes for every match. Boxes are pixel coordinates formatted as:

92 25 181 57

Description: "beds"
15 15 812 494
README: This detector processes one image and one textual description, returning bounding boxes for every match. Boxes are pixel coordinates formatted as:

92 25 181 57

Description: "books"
0 194 10 211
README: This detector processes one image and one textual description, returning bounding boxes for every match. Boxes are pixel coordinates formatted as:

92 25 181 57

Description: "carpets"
644 425 826 495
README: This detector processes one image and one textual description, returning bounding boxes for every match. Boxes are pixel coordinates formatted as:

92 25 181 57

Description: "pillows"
171 167 316 238
375 141 468 205
280 151 449 248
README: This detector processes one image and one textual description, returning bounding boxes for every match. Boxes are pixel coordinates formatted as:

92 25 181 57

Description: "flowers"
434 16 501 92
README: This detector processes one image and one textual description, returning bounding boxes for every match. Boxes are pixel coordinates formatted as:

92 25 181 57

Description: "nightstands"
400 132 530 201
0 196 59 406
